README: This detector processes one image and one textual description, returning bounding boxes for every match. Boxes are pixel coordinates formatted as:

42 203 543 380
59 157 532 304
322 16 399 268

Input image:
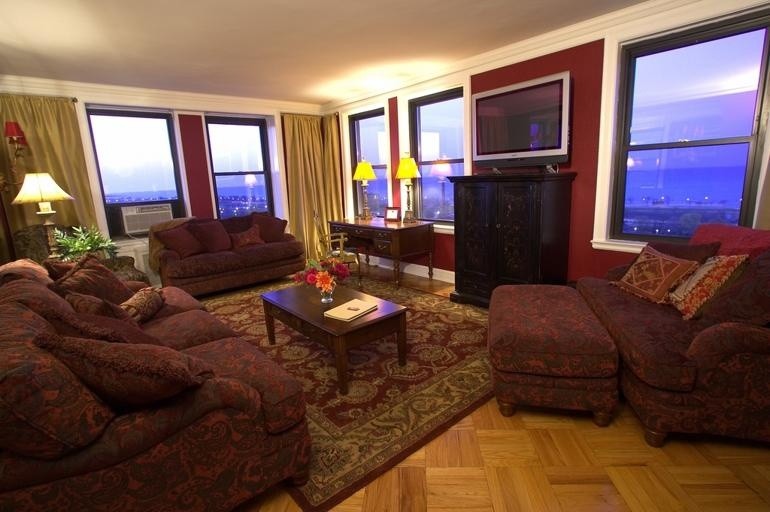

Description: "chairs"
312 209 362 288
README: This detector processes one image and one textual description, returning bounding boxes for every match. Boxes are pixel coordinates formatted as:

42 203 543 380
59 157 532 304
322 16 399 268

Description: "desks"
327 216 436 286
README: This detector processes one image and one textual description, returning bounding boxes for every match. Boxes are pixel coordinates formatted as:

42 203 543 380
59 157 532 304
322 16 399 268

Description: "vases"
321 291 334 304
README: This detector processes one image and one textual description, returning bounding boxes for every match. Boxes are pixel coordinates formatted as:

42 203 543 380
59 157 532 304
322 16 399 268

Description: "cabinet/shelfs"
446 172 578 308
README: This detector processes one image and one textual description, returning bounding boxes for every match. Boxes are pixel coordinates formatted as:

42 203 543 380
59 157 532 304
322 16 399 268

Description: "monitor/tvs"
471 70 571 173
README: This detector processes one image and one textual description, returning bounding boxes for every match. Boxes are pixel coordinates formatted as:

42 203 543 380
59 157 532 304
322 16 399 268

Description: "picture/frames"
384 207 401 222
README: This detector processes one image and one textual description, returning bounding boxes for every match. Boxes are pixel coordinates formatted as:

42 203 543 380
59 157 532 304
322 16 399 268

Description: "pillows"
669 254 751 320
33 329 215 403
46 253 135 305
38 307 165 345
187 220 233 253
618 244 701 304
664 247 770 358
616 240 721 292
60 288 140 329
250 211 283 242
42 259 73 280
153 224 208 259
228 223 266 250
119 288 166 325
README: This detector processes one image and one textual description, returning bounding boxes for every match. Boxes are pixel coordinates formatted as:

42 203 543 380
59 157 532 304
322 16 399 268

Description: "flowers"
292 256 352 303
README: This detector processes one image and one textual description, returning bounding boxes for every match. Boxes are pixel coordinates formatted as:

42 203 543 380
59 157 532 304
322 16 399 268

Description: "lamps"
0 122 28 191
10 172 75 260
395 157 421 223
353 162 377 220
429 159 455 219
244 174 258 187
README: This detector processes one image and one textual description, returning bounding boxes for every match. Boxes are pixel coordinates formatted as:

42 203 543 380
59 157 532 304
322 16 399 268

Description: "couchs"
149 218 306 296
487 284 619 427
576 224 770 447
13 223 151 287
0 258 312 512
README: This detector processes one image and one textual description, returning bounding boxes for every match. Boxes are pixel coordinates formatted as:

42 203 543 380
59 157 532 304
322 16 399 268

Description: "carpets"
196 260 495 512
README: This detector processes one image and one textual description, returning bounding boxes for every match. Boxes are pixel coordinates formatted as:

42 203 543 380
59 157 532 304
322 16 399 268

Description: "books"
324 300 377 322
